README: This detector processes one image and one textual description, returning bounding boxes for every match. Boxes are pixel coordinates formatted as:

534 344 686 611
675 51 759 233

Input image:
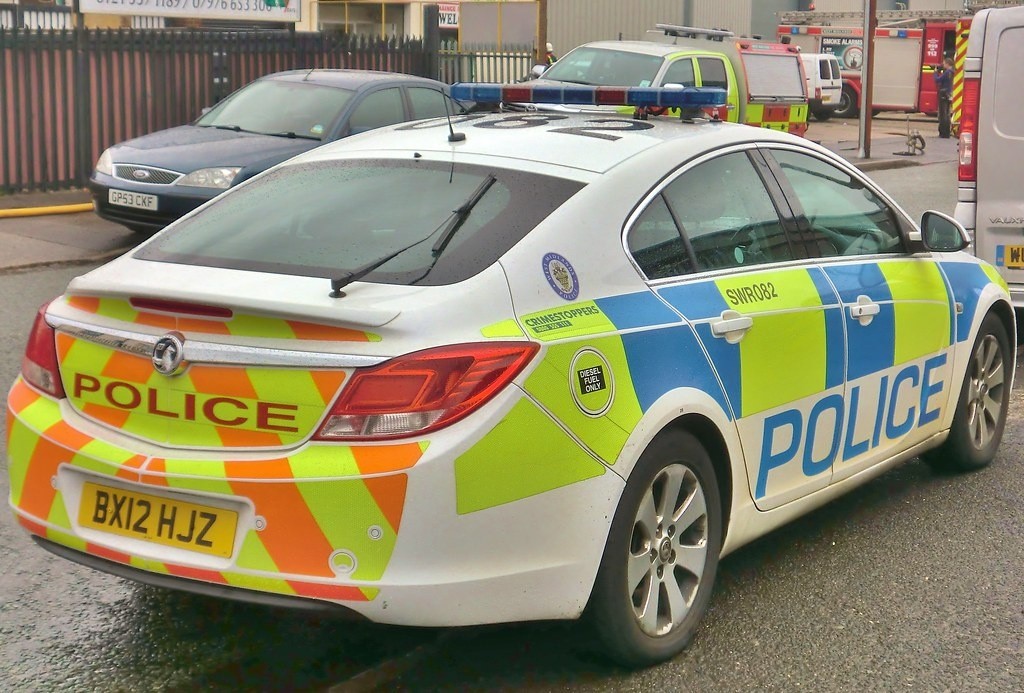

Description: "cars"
5 82 1018 668
88 41 492 233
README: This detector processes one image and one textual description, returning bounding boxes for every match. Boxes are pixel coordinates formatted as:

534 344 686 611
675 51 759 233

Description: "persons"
933 57 954 138
544 43 557 65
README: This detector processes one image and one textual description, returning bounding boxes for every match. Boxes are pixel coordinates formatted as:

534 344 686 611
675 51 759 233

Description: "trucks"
500 23 808 138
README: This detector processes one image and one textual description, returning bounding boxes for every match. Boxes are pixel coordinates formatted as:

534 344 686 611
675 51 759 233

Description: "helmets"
546 42 553 52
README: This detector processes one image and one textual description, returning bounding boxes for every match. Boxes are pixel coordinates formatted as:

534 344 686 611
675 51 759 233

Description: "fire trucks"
776 10 956 118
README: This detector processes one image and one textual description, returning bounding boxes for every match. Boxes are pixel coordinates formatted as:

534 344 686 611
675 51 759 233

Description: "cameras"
930 65 945 70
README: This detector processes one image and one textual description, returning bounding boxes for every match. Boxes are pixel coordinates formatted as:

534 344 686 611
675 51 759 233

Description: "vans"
953 6 1023 307
741 52 842 120
